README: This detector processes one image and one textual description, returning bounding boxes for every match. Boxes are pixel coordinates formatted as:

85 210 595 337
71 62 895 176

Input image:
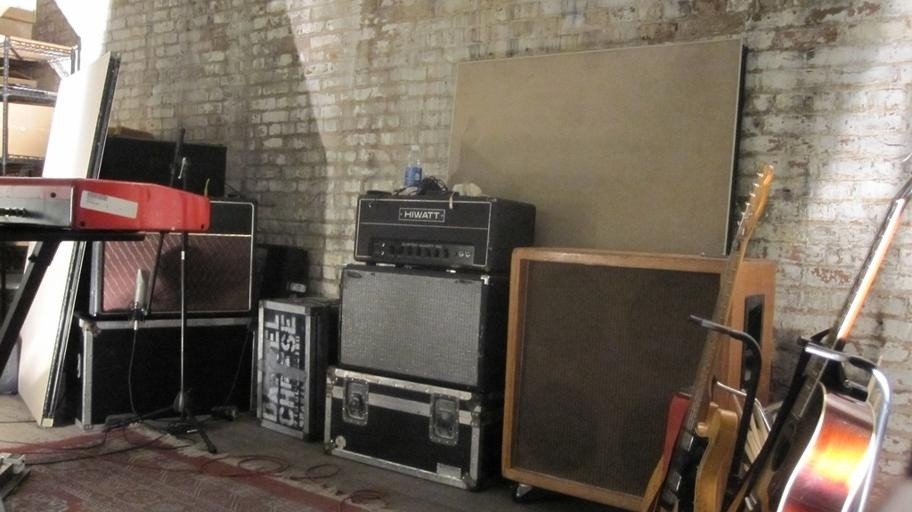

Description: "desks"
0 226 147 504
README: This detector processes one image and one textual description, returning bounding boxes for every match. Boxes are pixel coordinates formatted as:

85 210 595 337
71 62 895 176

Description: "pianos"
0 178 211 233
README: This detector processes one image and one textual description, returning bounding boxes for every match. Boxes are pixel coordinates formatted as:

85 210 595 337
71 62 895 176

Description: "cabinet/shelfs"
0 35 80 176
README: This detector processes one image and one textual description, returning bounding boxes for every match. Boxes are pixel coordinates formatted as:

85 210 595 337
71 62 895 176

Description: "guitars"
727 178 912 511
639 164 774 512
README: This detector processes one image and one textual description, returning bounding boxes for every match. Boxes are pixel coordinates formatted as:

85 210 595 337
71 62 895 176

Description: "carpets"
0 421 389 512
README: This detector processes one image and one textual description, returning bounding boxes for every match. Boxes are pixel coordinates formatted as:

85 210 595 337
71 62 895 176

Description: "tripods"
100 126 235 454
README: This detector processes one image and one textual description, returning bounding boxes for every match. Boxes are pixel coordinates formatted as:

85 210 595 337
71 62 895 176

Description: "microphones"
131 267 150 333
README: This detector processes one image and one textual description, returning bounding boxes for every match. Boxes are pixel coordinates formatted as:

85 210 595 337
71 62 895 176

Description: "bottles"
404 144 423 198
